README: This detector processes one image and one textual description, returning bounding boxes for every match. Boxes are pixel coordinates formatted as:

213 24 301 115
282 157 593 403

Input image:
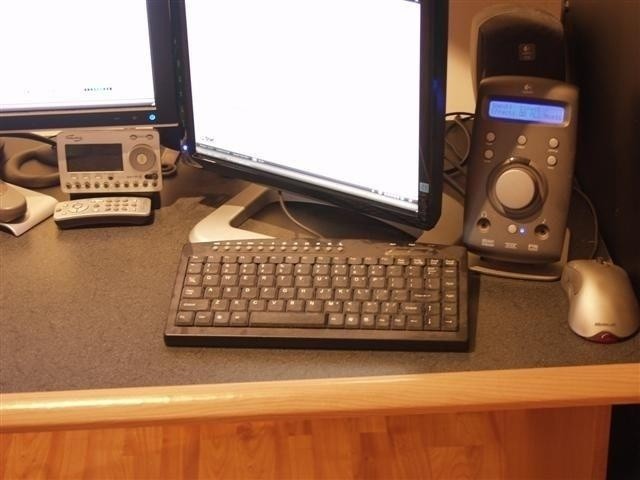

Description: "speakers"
469 4 568 108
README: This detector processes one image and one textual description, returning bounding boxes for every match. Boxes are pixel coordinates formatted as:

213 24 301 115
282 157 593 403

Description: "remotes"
52 196 155 228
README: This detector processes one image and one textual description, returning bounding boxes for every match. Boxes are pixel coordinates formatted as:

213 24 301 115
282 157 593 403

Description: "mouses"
560 254 639 344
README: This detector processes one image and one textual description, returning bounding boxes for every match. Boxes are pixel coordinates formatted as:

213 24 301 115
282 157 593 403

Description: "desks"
1 138 640 480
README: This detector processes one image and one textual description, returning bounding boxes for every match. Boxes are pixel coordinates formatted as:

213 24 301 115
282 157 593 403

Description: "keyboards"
161 240 472 353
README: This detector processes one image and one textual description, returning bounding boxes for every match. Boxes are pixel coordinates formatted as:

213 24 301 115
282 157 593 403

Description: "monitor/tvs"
165 0 466 245
0 0 181 186
